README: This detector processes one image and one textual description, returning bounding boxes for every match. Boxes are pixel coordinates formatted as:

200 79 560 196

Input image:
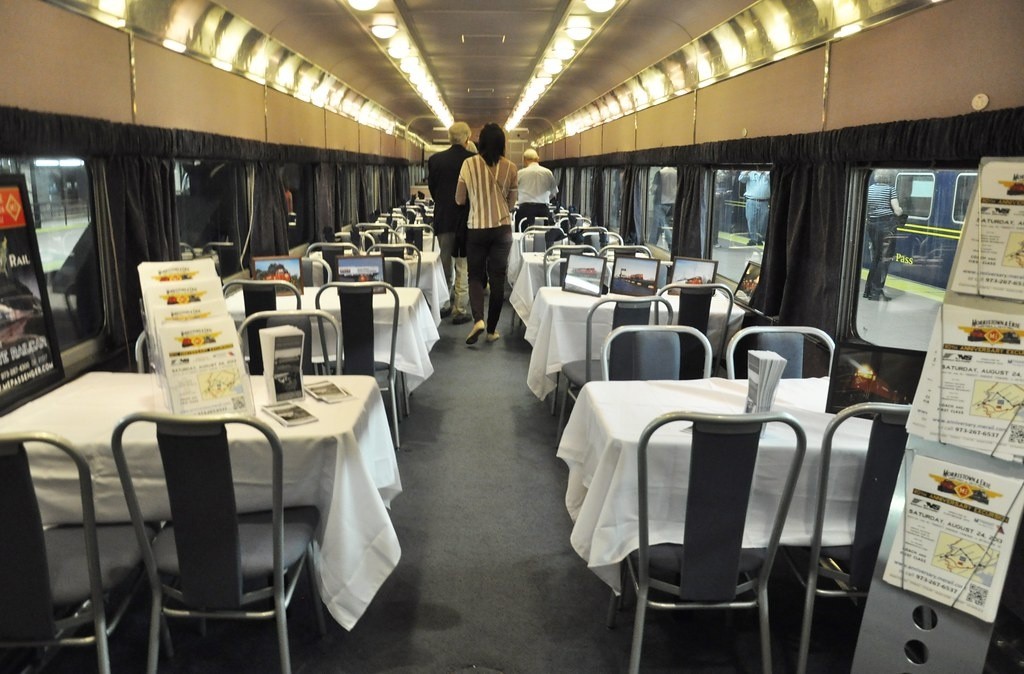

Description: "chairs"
601 324 713 382
606 410 808 674
654 282 734 380
795 401 913 674
237 310 344 377
300 195 654 289
726 326 836 380
222 279 302 375
0 430 176 673
553 296 674 449
314 281 403 451
110 412 330 674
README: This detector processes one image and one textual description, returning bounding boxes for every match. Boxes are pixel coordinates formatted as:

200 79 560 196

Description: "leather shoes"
488 331 500 343
464 320 485 345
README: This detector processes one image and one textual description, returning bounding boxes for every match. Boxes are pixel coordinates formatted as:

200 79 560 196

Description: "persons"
737 168 771 248
609 165 724 252
455 122 519 345
426 119 479 326
862 169 904 302
511 147 560 232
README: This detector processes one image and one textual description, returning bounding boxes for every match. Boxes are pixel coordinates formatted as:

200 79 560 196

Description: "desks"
580 377 874 612
540 285 745 417
224 286 422 418
0 372 381 634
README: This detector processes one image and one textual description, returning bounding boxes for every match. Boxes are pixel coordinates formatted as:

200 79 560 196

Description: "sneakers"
861 285 873 298
869 288 891 301
451 313 473 325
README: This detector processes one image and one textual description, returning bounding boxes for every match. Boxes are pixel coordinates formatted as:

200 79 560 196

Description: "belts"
746 198 770 202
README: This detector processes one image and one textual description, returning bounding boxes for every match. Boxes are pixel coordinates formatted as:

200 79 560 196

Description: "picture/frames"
610 253 661 298
733 260 769 316
825 341 927 421
666 255 719 297
250 255 304 297
562 252 607 298
0 173 67 418
